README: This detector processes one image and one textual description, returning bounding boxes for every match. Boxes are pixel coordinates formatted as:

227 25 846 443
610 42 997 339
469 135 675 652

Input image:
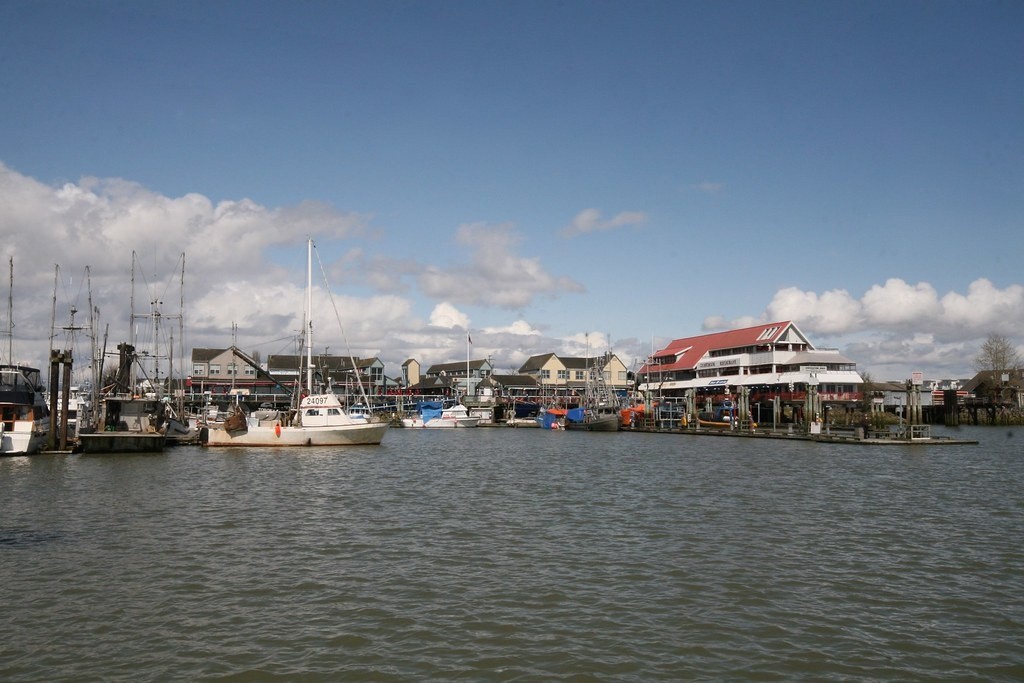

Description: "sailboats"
0 234 388 455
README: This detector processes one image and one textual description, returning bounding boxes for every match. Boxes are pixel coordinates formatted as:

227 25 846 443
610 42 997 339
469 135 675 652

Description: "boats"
401 403 480 428
506 404 756 432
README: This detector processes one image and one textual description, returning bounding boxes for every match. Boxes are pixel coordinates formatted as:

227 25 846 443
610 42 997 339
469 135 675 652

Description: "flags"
469 335 473 345
